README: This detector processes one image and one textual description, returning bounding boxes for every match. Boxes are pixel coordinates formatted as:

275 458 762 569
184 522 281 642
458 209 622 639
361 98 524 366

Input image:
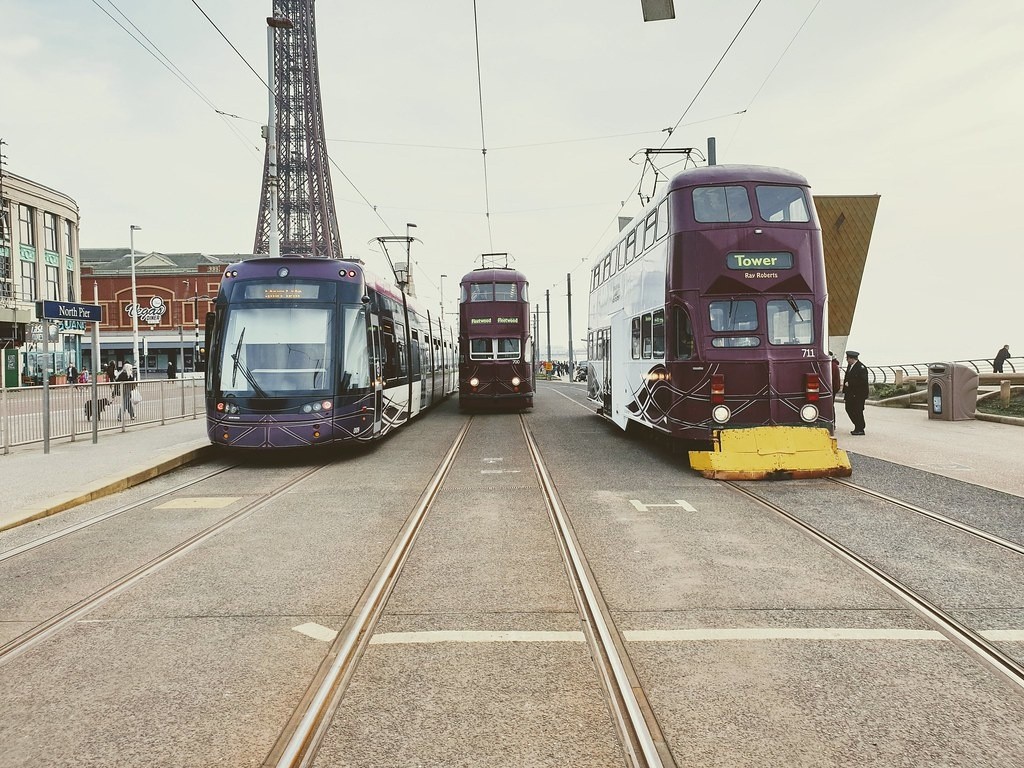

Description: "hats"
846 351 860 358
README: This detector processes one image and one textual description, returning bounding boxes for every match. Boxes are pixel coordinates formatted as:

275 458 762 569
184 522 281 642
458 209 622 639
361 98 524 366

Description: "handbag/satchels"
131 389 142 405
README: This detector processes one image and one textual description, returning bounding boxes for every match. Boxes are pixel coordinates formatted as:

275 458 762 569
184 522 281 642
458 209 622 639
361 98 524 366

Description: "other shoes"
850 429 865 435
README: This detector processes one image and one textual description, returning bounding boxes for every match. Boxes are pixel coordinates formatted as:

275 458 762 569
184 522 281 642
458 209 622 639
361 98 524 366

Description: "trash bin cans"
927 362 979 422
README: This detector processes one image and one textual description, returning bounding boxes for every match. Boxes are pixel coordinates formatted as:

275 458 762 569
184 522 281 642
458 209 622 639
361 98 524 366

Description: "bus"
584 135 838 450
453 253 537 408
203 249 458 450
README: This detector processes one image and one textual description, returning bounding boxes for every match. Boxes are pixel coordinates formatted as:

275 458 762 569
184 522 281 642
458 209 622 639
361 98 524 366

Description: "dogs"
84 398 110 422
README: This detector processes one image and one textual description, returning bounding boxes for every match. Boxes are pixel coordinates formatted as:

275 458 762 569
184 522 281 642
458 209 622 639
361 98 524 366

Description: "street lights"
129 223 141 383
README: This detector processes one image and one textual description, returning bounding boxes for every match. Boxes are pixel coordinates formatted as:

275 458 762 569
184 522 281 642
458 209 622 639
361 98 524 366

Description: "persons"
842 351 869 435
36 365 42 373
829 352 841 430
106 360 116 390
112 363 137 422
166 362 175 383
79 366 88 392
539 361 588 382
993 345 1011 372
67 363 78 391
